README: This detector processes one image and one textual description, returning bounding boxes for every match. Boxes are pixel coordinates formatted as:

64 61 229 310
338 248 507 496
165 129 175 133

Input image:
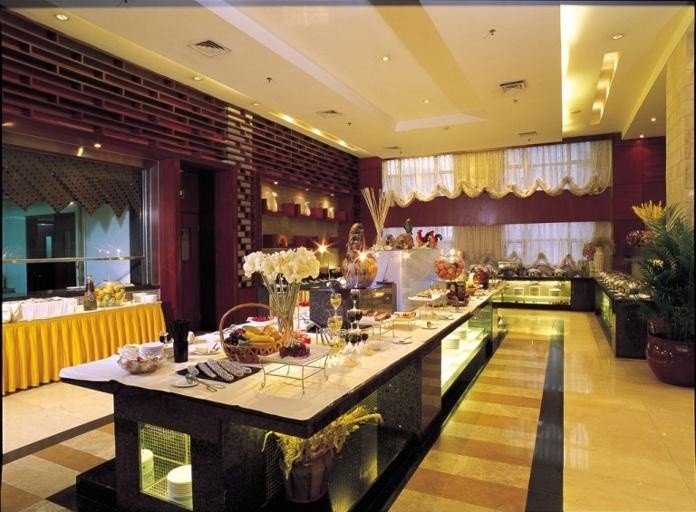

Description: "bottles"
83 273 97 311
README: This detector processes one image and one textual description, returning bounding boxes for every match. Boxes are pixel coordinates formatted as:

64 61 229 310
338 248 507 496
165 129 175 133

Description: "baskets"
219 302 290 365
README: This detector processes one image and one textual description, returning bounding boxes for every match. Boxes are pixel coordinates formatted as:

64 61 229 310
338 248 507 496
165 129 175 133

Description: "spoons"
399 335 412 342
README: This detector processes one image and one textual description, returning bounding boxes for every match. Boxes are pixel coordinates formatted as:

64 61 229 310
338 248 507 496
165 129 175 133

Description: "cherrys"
279 342 309 359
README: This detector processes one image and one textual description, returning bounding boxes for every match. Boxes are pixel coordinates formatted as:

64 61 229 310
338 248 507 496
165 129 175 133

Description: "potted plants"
629 203 696 388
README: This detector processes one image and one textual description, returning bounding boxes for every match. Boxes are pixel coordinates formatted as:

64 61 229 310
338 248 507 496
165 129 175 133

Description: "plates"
166 464 192 504
123 330 175 361
392 336 414 344
445 328 467 350
172 378 198 388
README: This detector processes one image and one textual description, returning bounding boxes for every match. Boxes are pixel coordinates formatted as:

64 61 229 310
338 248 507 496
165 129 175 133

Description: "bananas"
247 335 275 343
241 329 255 339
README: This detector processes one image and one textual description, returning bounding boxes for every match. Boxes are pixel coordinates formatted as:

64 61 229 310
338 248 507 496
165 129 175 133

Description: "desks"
2 297 163 397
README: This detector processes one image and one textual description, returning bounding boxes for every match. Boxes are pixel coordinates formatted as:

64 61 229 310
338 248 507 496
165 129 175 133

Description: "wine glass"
320 288 369 370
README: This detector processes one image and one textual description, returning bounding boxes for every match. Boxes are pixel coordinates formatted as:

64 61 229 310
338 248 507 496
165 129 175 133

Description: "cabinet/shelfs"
248 176 341 276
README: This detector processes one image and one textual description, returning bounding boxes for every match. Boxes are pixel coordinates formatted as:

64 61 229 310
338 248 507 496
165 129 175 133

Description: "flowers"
582 236 609 259
243 248 323 349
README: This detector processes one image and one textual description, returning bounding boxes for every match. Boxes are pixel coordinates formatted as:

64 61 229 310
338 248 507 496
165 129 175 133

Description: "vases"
267 285 299 349
588 246 605 273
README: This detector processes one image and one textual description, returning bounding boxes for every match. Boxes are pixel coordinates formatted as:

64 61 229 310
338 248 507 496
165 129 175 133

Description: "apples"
95 282 125 306
